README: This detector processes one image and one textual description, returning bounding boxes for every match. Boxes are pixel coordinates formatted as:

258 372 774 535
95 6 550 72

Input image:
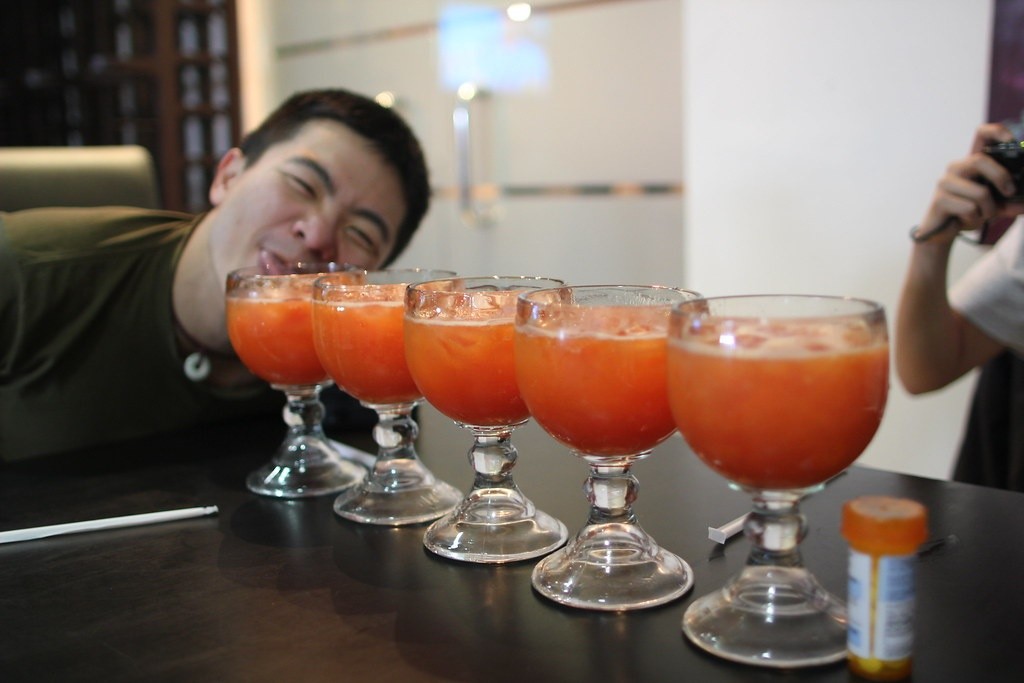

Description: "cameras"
974 137 1024 204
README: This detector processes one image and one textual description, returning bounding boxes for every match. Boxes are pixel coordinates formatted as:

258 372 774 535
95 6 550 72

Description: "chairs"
0 145 161 210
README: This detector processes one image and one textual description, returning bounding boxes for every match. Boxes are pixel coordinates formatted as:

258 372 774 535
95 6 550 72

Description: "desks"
0 395 1024 683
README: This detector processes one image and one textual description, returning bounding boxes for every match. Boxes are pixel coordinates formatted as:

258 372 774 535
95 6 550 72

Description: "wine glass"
312 271 464 527
668 294 890 668
403 275 571 565
514 285 711 612
224 262 368 499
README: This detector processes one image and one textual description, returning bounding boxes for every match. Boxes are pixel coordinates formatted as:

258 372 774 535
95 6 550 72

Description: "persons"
0 87 431 459
893 120 1024 396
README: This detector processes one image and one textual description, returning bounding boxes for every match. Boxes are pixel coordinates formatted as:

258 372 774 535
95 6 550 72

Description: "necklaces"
172 309 235 381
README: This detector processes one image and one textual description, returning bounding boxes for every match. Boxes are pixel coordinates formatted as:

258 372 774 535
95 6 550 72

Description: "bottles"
840 492 927 680
